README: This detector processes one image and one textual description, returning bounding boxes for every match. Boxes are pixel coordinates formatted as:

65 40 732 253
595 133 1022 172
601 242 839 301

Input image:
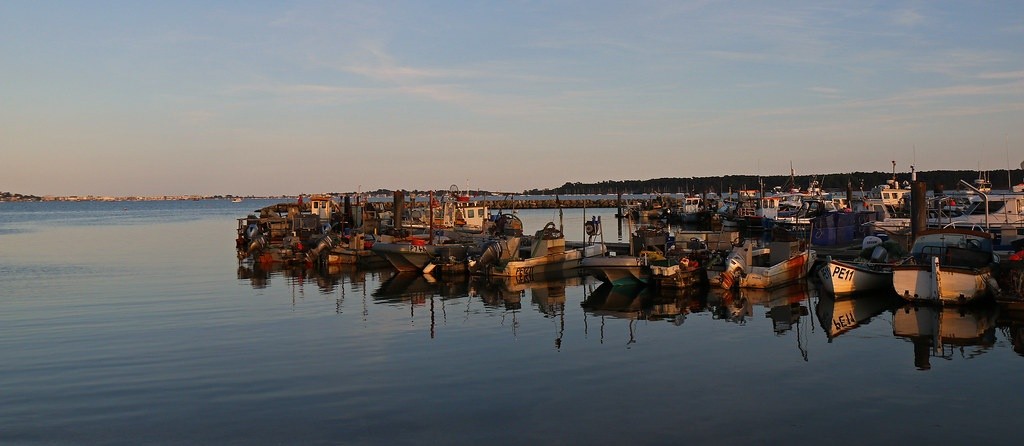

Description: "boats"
706 270 819 335
892 221 996 307
890 296 998 371
814 294 892 343
579 281 648 319
373 273 452 303
234 160 1024 290
818 254 893 300
639 284 707 327
